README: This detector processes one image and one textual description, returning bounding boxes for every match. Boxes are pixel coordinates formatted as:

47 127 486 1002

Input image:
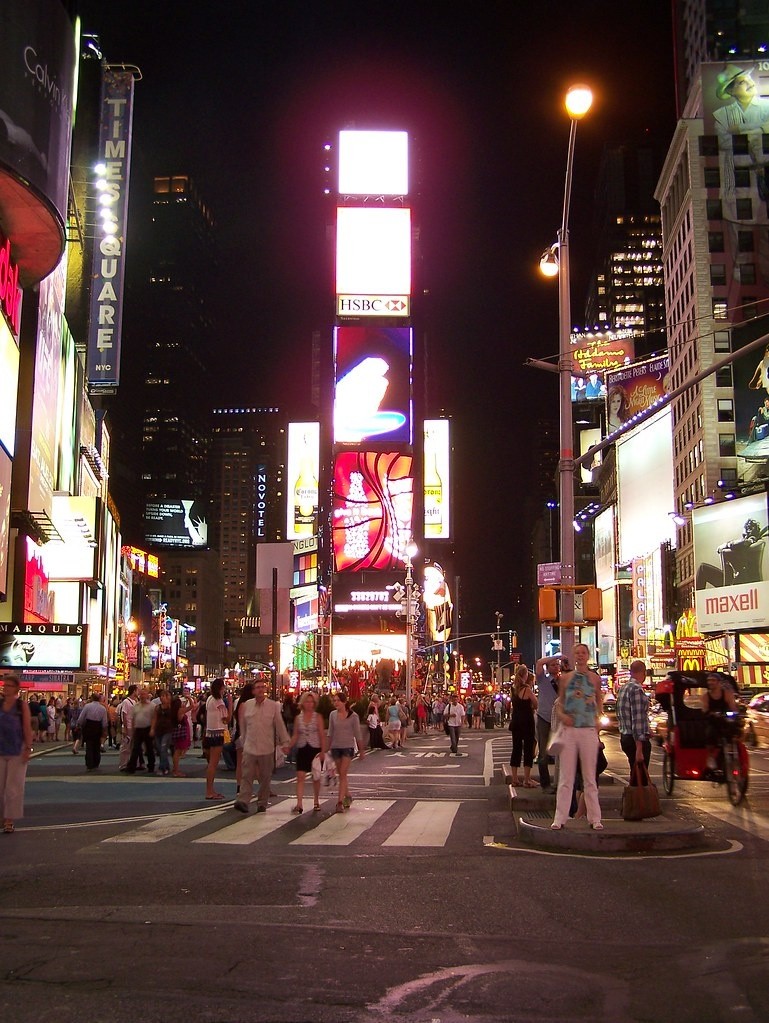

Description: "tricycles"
655 670 749 806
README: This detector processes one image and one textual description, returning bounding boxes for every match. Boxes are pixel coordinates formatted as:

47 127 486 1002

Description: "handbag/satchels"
444 722 450 735
545 723 565 756
46 718 51 726
398 707 407 721
621 759 661 821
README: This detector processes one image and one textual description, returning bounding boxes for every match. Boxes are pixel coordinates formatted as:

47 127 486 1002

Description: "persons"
1 634 36 666
747 344 769 442
608 386 632 437
574 374 607 401
0 643 769 834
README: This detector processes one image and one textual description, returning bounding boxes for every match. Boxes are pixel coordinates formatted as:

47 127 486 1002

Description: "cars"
744 691 769 746
601 701 619 731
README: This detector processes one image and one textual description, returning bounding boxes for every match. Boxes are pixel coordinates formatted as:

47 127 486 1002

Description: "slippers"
206 794 224 799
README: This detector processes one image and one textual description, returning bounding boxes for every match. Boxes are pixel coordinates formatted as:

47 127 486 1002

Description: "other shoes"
551 822 563 829
72 748 78 754
512 781 523 786
454 752 462 756
523 781 537 788
542 786 556 794
87 767 95 772
257 806 266 812
344 796 351 807
590 823 603 830
234 801 248 813
156 769 163 777
147 766 153 771
336 802 345 813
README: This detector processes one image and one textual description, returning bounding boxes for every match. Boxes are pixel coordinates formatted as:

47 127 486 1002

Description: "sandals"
313 803 321 811
4 821 15 833
292 806 303 814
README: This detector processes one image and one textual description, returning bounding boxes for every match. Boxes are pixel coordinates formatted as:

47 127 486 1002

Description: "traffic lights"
423 637 433 661
438 649 455 674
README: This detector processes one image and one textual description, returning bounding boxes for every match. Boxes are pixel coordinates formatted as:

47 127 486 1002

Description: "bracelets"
26 746 35 752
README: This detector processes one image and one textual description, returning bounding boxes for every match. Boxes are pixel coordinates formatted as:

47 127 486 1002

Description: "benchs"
654 670 739 747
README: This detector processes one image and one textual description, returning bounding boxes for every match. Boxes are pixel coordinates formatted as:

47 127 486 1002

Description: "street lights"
405 539 418 710
538 82 598 670
602 634 632 673
682 642 732 677
139 631 146 690
497 614 503 669
